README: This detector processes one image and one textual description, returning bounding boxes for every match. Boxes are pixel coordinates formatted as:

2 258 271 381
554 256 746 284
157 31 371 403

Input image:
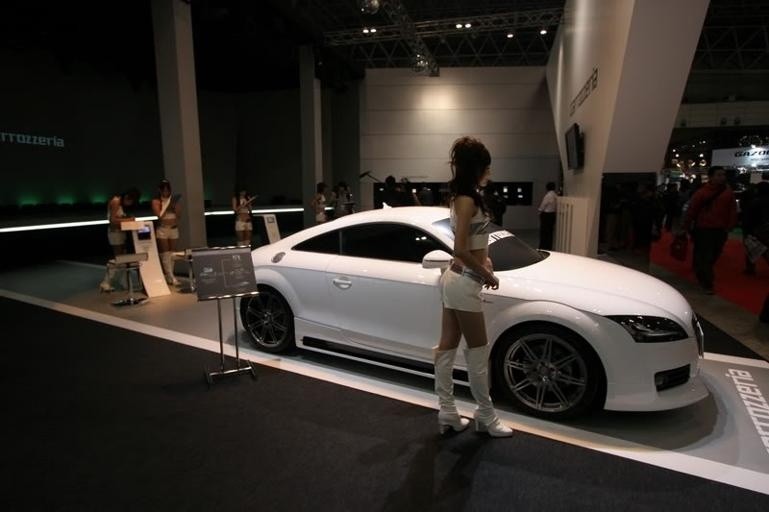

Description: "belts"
160 224 177 229
449 262 485 285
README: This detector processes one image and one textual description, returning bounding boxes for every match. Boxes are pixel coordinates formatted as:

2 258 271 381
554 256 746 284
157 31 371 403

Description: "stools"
171 249 195 294
107 251 148 307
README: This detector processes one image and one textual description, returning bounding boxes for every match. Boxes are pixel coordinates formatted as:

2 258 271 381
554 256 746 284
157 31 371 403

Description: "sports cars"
231 207 710 420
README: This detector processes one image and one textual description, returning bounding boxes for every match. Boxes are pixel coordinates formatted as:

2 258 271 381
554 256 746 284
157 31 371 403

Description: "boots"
99 260 117 292
432 345 470 434
162 251 181 287
119 269 128 290
462 340 513 437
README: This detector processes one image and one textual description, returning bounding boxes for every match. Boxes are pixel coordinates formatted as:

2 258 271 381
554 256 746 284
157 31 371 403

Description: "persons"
149 179 184 287
600 158 769 326
231 184 256 245
432 137 513 439
534 179 563 250
373 175 506 228
309 181 356 223
96 187 140 294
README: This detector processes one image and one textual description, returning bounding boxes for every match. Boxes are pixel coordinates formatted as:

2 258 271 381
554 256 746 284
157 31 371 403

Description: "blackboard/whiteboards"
191 245 261 302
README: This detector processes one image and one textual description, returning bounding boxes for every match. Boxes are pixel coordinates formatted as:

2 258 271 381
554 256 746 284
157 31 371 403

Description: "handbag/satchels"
670 230 688 258
742 235 767 264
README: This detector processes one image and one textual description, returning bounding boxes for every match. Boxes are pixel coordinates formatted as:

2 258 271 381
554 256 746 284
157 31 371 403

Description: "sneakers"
699 267 756 295
